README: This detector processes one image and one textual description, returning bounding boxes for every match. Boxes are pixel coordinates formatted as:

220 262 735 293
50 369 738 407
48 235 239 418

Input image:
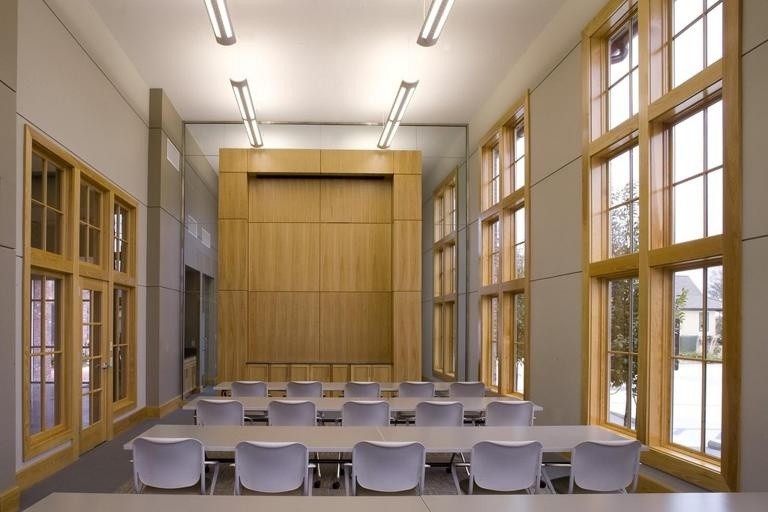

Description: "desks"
126 425 649 485
22 491 767 512
183 396 543 423
214 380 453 396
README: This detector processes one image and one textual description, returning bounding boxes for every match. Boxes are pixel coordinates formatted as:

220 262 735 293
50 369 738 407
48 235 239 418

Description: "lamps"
229 77 264 149
417 1 455 47
203 1 236 46
377 69 419 149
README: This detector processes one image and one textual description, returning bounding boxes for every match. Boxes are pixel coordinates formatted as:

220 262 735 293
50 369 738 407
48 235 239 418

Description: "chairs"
340 401 391 426
566 440 643 491
196 399 246 425
484 401 534 426
449 440 557 494
286 381 324 399
398 382 435 399
415 401 465 426
343 382 381 398
234 440 315 495
130 437 221 494
266 400 318 427
344 440 429 493
229 381 269 398
448 382 483 397
